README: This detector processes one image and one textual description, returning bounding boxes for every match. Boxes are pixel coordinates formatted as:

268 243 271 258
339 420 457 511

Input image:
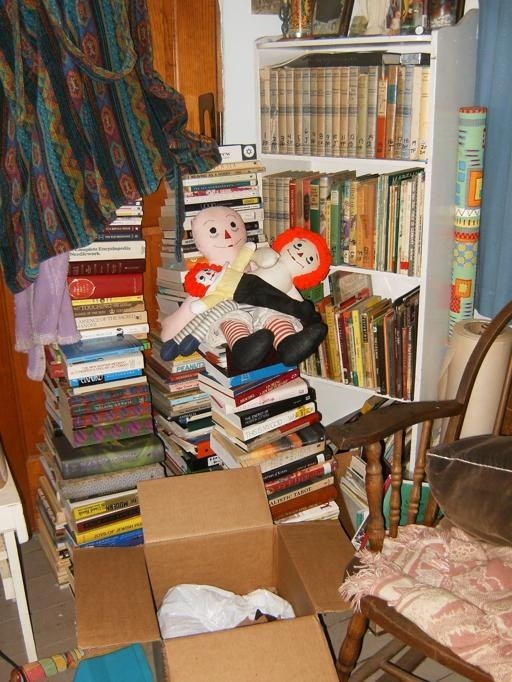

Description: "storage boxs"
72 463 356 681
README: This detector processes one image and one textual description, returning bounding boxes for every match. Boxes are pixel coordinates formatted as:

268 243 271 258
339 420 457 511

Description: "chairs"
324 300 512 681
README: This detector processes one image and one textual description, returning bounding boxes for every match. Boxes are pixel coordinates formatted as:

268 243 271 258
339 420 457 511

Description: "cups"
401 0 459 35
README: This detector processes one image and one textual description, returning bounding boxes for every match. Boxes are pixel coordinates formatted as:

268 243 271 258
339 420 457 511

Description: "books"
263 167 426 277
30 146 342 594
299 270 420 401
260 66 429 162
338 397 431 528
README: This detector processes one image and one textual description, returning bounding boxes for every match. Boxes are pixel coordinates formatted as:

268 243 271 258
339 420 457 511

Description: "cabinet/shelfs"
254 9 478 403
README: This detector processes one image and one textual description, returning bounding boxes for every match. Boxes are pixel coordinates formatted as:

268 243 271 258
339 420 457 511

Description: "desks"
0 455 39 662
